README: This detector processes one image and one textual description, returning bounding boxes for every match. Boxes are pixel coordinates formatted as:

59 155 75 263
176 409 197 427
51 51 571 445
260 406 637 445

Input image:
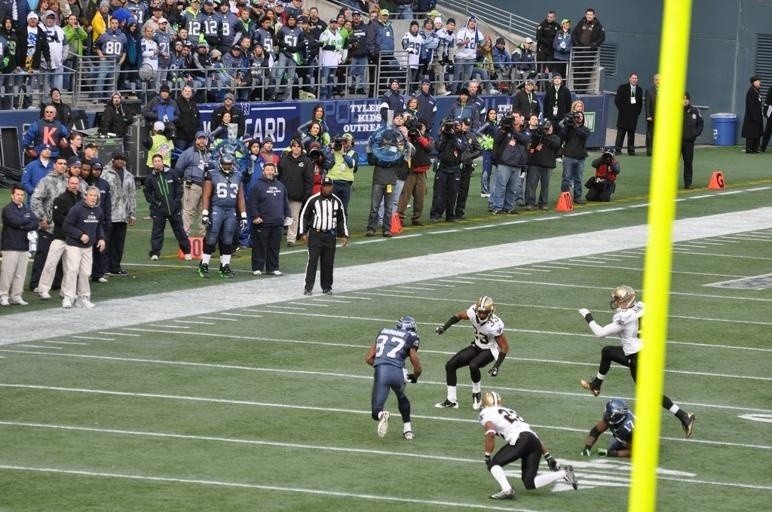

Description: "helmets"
397 317 417 332
476 296 494 324
482 391 501 408
611 285 636 309
219 144 235 175
604 399 628 427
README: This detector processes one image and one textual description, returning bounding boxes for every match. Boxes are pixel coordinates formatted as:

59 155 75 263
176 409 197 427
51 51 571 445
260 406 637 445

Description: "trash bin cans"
710 113 738 146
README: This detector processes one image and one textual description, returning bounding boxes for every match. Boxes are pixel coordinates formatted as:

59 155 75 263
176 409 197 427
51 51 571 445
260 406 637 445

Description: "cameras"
310 149 320 160
602 151 613 162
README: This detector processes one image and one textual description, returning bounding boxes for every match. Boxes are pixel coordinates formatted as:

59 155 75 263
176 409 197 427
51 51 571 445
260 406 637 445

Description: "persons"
758 86 772 152
581 399 635 457
578 286 694 438
365 316 421 440
741 76 764 153
435 296 508 410
1 1 704 309
479 392 577 499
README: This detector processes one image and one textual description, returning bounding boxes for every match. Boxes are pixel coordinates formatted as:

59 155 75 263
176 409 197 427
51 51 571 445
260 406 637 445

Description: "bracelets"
484 452 492 461
544 452 552 460
585 313 594 324
585 445 591 450
607 450 617 456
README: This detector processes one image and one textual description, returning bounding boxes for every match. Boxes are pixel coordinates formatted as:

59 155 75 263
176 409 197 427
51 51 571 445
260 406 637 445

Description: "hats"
321 177 333 184
36 143 104 170
524 37 534 44
154 121 165 132
27 0 389 58
160 85 170 93
261 138 273 146
196 130 207 138
243 133 252 144
427 9 442 24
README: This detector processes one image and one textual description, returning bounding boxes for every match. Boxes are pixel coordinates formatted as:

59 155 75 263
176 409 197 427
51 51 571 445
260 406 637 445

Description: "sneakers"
682 412 694 438
366 209 466 237
199 260 211 278
580 380 601 396
253 270 262 276
266 269 282 275
378 411 390 438
0 287 94 308
563 465 577 489
575 198 585 204
435 399 459 408
481 192 549 215
219 263 234 277
184 253 192 260
323 287 332 295
303 285 312 296
472 392 482 410
403 431 414 439
490 489 515 498
96 267 128 281
152 254 159 260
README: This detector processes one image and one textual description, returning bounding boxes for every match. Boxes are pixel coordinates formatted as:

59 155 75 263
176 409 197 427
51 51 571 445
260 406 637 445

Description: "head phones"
351 137 354 147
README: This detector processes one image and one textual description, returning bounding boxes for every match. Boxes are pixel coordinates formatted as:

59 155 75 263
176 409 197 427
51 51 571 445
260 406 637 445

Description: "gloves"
581 444 592 456
489 364 498 376
435 324 445 335
407 373 417 384
484 452 491 464
544 452 560 471
596 448 617 457
578 307 593 323
